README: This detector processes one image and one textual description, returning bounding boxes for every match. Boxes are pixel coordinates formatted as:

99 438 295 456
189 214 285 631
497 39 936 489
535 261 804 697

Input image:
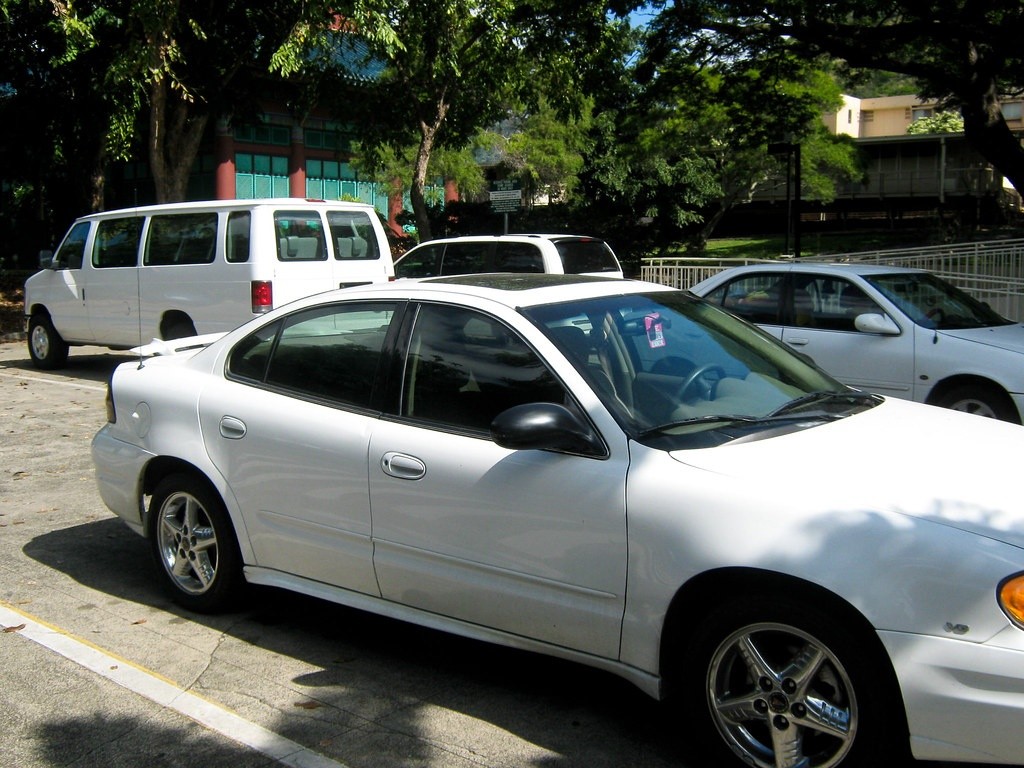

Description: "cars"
393 235 642 349
626 262 1023 426
90 270 1023 768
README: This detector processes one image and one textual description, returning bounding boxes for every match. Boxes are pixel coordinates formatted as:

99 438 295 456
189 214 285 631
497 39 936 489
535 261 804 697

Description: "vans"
24 197 397 372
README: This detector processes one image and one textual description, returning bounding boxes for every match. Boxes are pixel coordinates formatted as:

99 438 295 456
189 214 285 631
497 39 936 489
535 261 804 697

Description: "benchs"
280 236 317 259
151 235 216 262
337 238 373 258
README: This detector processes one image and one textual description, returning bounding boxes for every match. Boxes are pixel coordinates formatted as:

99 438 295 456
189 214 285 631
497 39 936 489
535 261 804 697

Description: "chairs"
793 289 812 326
531 326 633 418
840 286 884 317
